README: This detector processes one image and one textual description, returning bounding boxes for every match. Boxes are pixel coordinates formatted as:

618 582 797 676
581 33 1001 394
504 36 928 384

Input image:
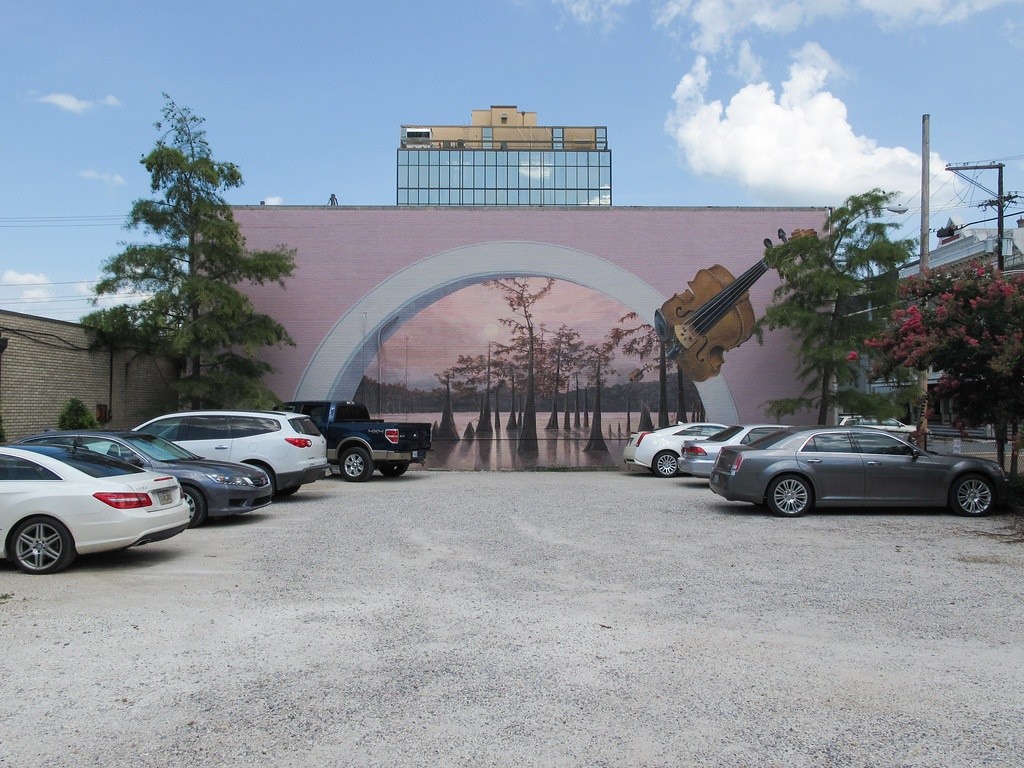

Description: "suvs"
80 408 333 498
836 415 933 446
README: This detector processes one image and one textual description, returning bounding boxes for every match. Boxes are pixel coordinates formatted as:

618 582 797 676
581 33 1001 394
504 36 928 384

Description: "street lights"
995 211 1024 272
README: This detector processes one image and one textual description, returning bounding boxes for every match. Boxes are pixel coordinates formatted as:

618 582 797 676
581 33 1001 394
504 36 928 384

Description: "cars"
675 425 824 480
711 425 1009 518
623 421 730 478
0 429 273 529
0 445 191 575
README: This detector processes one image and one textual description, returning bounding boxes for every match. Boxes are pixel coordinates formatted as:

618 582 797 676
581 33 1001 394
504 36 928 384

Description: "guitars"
653 225 821 385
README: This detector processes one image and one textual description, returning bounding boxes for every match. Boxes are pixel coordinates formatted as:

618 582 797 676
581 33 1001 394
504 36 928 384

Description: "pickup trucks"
272 401 435 482
